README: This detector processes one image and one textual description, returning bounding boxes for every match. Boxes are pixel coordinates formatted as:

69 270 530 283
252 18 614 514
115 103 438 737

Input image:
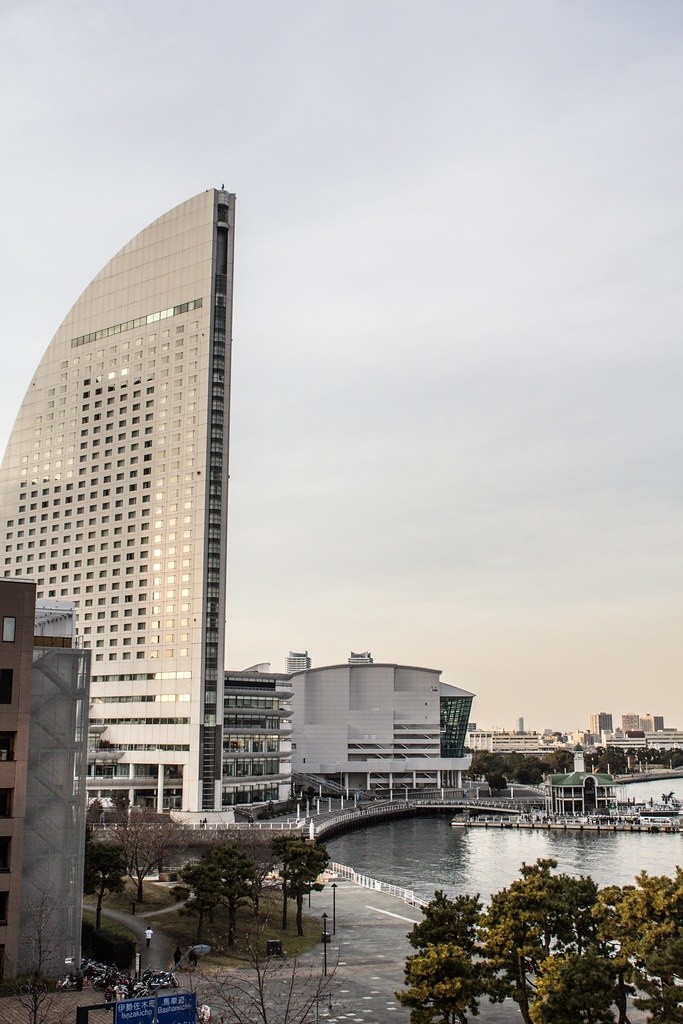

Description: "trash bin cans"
267 940 282 955
321 932 331 943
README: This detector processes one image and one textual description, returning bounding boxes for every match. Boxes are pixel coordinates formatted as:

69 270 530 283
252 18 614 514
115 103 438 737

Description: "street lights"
321 913 329 976
330 882 338 934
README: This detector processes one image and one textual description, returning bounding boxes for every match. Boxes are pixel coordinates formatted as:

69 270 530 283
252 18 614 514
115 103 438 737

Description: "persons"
104 983 114 1012
74 967 84 991
173 946 182 971
200 818 208 829
144 926 153 950
187 942 197 967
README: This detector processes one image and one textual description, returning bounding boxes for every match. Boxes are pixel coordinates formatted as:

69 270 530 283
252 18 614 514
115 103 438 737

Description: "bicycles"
20 975 48 997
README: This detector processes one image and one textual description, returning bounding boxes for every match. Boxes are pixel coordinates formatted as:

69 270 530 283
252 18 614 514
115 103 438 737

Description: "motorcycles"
59 955 180 1004
